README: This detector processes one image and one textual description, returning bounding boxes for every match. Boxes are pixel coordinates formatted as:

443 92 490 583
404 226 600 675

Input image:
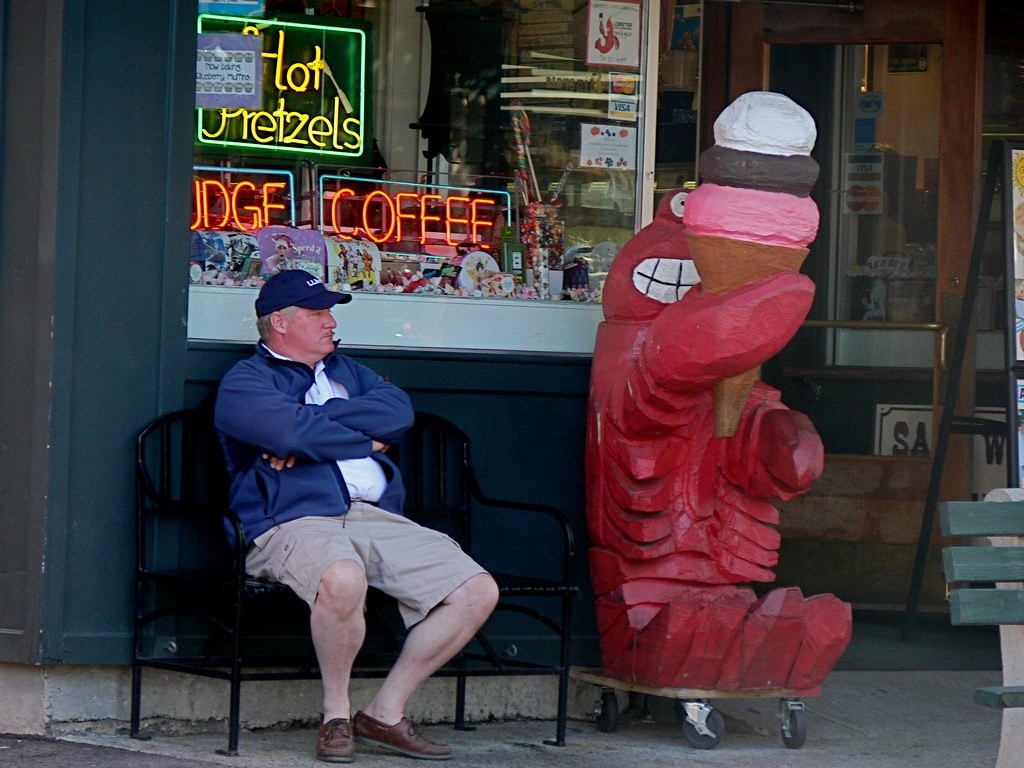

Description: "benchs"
938 501 1024 708
128 407 582 756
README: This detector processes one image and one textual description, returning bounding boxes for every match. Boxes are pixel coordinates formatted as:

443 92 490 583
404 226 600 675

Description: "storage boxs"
501 219 564 295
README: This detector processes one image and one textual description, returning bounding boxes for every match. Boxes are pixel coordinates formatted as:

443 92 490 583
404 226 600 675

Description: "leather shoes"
352 710 451 759
316 713 354 762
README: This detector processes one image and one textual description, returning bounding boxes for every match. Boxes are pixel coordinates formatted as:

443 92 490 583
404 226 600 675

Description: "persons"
338 243 373 281
260 238 290 277
215 269 501 762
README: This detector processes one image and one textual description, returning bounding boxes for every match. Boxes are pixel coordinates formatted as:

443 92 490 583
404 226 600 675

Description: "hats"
254 269 352 319
